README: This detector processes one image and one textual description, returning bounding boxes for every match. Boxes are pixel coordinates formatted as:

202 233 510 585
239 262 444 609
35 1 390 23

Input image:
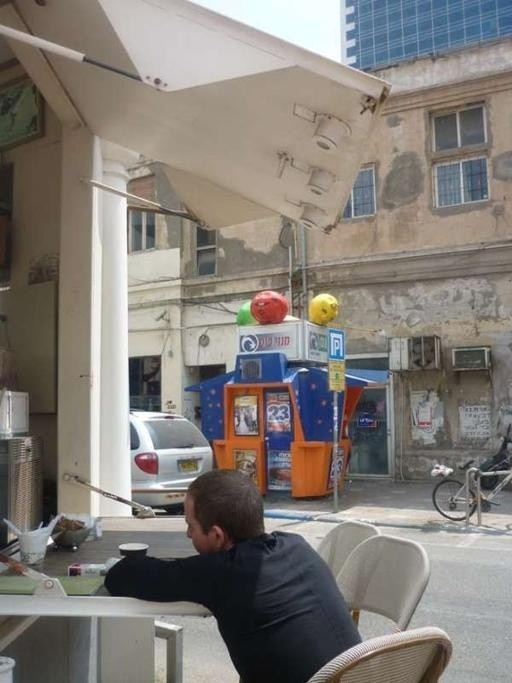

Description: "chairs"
336 534 431 632
307 626 452 682
312 520 382 628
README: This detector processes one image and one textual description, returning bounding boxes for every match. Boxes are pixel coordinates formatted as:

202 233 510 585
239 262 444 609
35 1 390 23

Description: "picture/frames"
0 57 45 153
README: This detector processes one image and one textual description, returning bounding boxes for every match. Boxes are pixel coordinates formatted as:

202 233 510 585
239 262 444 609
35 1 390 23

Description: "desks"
0 525 216 683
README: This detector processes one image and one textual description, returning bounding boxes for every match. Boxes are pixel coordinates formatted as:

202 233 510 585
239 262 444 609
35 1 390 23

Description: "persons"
104 469 363 683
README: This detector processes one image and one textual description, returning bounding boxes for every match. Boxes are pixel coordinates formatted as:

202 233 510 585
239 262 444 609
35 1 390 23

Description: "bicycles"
432 459 512 520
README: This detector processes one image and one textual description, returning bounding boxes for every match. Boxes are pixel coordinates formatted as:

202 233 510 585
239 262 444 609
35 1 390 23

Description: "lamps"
300 206 324 232
306 169 335 196
312 116 350 154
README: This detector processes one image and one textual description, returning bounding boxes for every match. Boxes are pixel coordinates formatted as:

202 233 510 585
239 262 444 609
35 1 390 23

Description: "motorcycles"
478 422 512 489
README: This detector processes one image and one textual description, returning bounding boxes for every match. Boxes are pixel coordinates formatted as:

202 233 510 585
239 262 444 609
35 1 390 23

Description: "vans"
127 410 213 507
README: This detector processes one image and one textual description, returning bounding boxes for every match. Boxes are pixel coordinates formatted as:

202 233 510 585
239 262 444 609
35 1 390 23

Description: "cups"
118 543 149 557
16 532 50 564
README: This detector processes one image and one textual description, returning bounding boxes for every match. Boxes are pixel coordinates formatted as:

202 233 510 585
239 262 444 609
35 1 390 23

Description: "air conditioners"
389 335 441 371
452 347 491 372
